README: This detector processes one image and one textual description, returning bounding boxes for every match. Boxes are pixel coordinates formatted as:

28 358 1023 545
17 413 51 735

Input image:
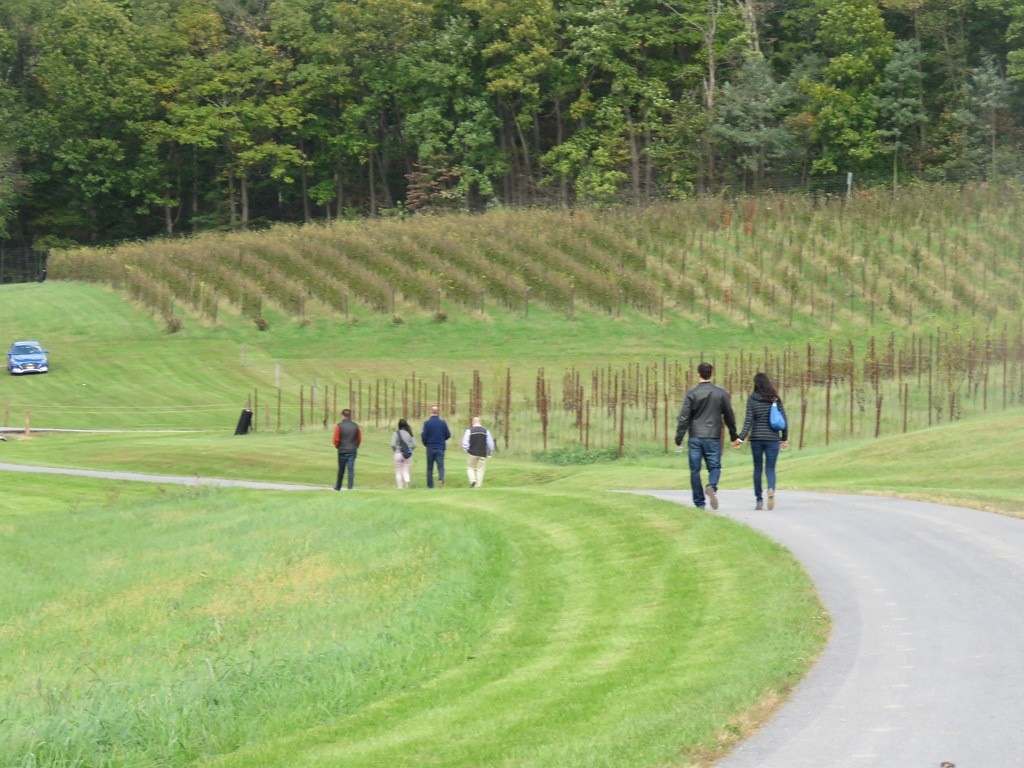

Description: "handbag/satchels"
397 431 412 459
769 395 785 430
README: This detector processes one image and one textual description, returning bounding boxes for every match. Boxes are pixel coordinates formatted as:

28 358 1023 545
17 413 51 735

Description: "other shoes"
706 485 719 510
470 481 476 488
767 488 776 510
754 501 763 510
439 480 444 487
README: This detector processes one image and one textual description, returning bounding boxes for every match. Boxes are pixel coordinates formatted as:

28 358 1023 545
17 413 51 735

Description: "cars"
5 341 50 375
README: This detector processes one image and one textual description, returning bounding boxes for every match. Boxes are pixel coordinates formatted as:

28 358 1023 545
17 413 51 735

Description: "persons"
421 405 451 488
462 417 494 487
734 373 790 511
333 409 362 491
391 419 416 490
675 363 741 511
